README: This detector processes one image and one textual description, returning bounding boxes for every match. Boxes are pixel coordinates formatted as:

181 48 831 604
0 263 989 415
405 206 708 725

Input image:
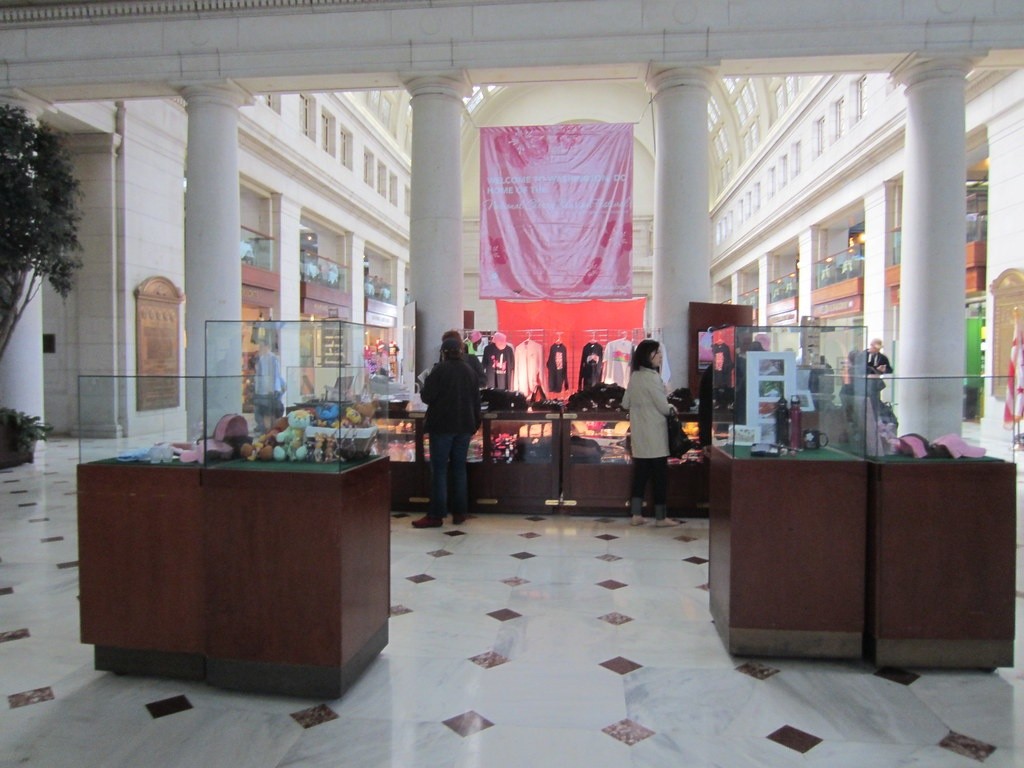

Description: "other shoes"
656 518 679 528
631 515 648 525
453 514 465 525
412 514 443 528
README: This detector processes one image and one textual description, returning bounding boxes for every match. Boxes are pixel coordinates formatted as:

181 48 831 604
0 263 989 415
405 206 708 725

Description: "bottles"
777 398 789 447
789 395 802 450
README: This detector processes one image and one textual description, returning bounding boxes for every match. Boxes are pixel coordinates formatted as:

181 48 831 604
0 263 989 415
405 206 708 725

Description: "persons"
374 339 400 382
784 344 886 420
621 339 681 528
858 338 894 398
697 334 746 448
712 339 735 388
248 339 288 433
482 332 515 392
409 330 488 526
470 331 488 363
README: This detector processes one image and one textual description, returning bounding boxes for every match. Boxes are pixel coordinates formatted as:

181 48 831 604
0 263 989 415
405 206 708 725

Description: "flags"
1001 322 1024 429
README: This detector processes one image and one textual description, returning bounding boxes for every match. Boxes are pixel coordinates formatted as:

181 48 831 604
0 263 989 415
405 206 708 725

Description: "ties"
870 354 875 366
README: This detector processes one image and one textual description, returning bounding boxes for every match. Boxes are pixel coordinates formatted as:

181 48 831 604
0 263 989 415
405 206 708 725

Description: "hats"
871 339 884 350
896 434 987 459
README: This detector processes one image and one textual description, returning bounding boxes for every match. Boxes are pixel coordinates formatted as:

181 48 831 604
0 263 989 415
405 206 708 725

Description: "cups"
804 429 829 449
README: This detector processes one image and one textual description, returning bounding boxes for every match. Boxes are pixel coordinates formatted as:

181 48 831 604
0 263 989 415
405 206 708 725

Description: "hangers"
710 331 732 351
458 328 641 352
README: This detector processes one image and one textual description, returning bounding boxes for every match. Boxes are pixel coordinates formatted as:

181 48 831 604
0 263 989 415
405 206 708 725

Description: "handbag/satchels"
667 409 690 457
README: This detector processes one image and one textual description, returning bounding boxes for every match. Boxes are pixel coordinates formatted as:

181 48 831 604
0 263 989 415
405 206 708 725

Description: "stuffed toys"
301 399 378 428
241 408 334 464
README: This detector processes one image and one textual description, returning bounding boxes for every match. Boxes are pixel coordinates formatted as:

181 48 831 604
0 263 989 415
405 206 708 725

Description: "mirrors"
709 325 735 404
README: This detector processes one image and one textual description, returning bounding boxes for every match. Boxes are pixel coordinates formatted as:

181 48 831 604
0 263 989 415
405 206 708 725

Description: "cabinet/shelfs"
711 324 867 670
372 412 560 519
77 321 392 701
811 373 1019 673
561 408 736 516
